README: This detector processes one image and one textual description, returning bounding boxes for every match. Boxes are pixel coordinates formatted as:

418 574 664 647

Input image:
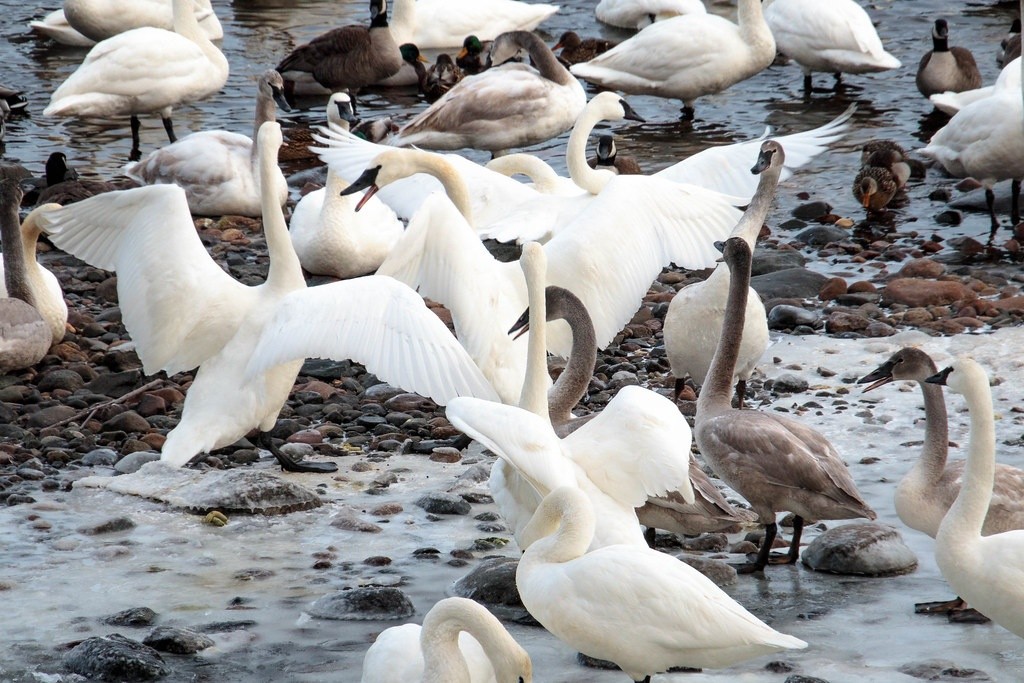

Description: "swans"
292 91 862 418
360 598 531 683
42 0 232 162
0 177 52 374
0 203 69 346
596 0 707 30
515 486 808 682
924 358 1024 637
382 32 585 161
857 348 1023 624
29 0 224 48
117 68 293 217
760 0 903 89
565 0 779 120
694 237 878 575
448 237 692 555
35 119 501 470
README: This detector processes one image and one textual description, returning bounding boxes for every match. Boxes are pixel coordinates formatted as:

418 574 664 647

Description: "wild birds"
853 139 926 212
996 18 1022 69
550 33 620 64
911 56 1022 238
372 42 429 88
277 0 403 120
455 34 524 73
916 19 982 98
423 53 465 105
